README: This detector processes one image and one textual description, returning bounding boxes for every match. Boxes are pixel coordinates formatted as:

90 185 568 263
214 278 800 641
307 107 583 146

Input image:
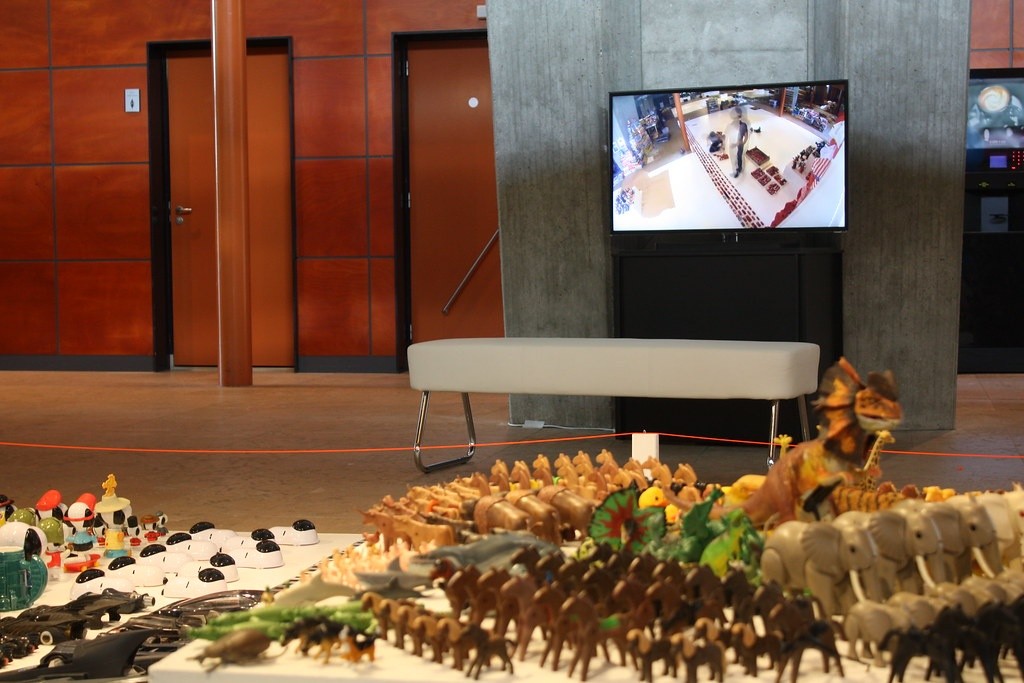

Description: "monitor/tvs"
966 66 1024 148
609 80 849 237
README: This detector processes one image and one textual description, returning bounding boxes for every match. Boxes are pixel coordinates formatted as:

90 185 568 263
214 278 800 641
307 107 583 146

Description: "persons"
724 107 748 178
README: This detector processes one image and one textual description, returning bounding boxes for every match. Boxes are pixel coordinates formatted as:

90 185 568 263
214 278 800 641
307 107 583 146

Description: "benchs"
407 338 821 474
